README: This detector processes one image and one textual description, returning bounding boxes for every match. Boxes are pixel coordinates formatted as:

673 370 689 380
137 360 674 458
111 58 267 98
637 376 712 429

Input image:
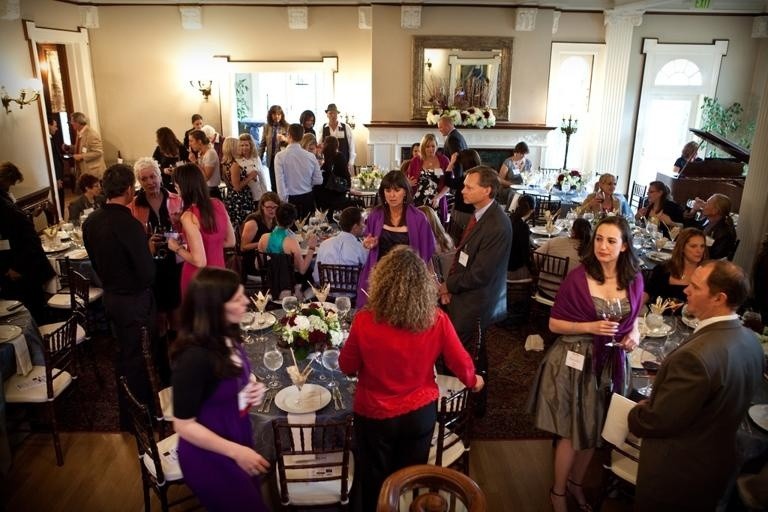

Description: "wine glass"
168 165 176 185
596 192 606 211
546 210 680 258
251 163 258 182
143 221 179 261
667 296 684 325
240 311 269 346
352 162 382 192
630 313 666 362
53 218 84 251
637 334 683 398
260 292 354 402
599 298 626 348
295 213 345 250
683 303 698 327
521 169 594 198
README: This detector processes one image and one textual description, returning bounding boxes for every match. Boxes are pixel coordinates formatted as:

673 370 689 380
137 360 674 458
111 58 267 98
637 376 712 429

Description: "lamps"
424 57 432 71
0 79 41 113
189 80 213 100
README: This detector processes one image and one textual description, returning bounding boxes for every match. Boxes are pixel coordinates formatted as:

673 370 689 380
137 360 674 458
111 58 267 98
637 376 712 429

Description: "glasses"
263 205 278 210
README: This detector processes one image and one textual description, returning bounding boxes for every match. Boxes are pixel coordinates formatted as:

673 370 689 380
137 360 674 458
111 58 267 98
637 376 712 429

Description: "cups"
687 199 705 210
705 234 715 247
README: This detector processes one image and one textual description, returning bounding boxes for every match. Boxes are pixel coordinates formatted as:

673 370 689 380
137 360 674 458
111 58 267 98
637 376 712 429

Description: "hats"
325 104 340 114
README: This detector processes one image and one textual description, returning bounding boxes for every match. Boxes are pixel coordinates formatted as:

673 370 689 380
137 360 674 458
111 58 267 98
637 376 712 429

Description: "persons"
1 103 766 438
523 215 645 511
165 265 274 511
336 244 487 511
626 257 766 512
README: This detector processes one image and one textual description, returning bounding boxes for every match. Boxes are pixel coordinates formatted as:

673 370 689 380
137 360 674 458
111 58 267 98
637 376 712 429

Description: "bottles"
116 150 123 164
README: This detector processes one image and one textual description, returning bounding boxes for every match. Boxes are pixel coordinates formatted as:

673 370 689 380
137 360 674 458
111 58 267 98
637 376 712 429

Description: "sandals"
549 489 567 512
565 480 592 511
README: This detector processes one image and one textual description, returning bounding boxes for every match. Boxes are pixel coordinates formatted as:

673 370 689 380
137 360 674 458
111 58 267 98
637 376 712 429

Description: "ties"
461 214 476 244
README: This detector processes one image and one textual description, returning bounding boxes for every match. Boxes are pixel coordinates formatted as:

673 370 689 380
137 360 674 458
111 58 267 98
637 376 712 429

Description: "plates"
572 197 585 203
748 404 767 433
636 258 645 266
681 316 700 329
239 312 275 331
528 225 560 246
35 227 90 259
1 301 25 343
275 382 332 413
647 251 672 263
629 348 657 369
511 185 539 195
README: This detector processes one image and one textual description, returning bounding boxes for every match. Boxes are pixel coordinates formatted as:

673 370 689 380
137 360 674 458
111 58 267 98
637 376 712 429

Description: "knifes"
328 382 348 411
259 385 276 415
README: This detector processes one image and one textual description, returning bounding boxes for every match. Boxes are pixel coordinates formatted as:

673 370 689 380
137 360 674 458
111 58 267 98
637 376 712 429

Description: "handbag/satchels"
324 174 349 192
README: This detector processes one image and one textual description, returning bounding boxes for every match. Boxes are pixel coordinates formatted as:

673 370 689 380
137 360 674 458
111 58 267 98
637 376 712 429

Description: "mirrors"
409 34 514 121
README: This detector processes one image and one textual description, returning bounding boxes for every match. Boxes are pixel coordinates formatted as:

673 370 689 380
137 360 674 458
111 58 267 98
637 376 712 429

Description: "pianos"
656 129 750 215
